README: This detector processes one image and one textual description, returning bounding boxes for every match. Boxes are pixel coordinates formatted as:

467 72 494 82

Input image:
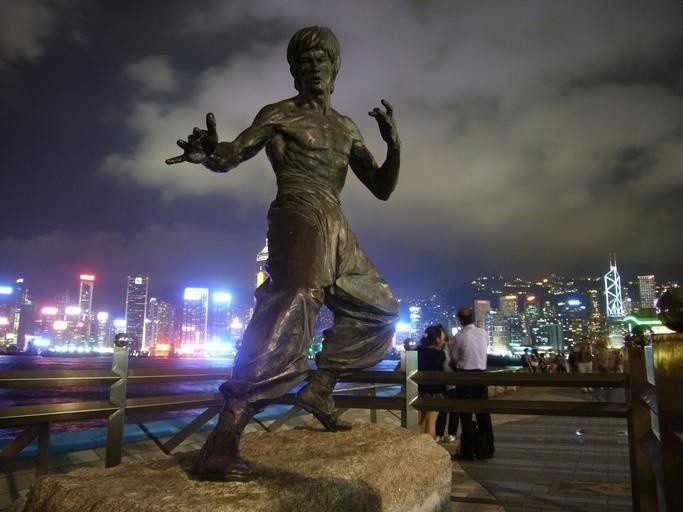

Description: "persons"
164 26 400 483
521 347 568 374
417 308 496 460
574 339 624 392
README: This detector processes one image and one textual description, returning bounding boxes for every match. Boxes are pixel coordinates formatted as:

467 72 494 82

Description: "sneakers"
434 434 456 443
